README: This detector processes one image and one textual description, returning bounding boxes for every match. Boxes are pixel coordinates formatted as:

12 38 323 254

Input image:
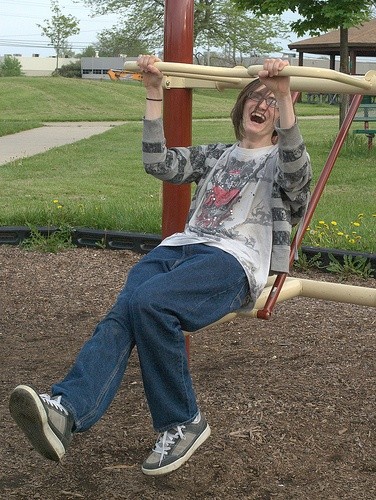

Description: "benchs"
353 103 376 149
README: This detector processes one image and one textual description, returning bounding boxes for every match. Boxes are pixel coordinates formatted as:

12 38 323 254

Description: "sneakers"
9 384 75 462
142 411 211 476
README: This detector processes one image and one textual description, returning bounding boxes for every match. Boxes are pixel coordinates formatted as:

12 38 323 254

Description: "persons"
8 55 313 477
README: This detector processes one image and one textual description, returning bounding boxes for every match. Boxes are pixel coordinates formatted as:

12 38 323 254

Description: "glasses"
248 92 279 108
